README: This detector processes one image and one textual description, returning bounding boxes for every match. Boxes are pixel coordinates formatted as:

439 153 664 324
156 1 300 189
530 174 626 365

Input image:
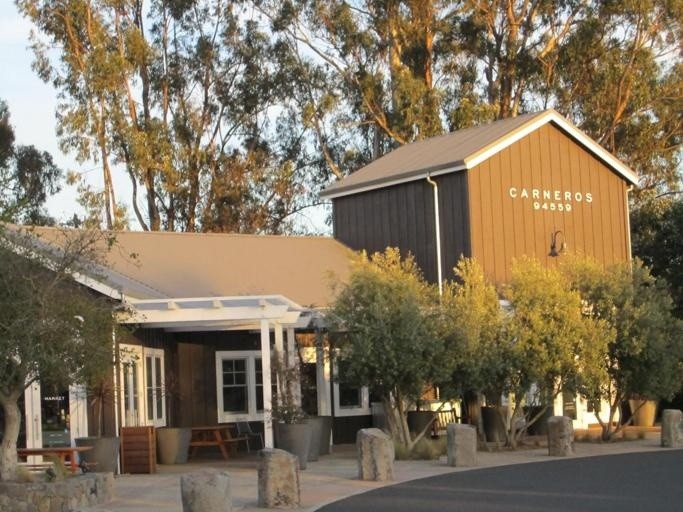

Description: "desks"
189 426 236 460
16 446 92 476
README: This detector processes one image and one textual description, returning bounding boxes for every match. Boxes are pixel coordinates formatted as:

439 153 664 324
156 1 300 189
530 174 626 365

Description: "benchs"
22 463 97 477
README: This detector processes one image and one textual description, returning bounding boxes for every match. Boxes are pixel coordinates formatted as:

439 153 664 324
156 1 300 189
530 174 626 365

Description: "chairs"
236 416 264 453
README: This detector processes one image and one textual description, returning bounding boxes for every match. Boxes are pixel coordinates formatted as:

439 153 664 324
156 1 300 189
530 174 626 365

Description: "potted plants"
269 349 313 470
73 377 121 479
149 375 192 465
